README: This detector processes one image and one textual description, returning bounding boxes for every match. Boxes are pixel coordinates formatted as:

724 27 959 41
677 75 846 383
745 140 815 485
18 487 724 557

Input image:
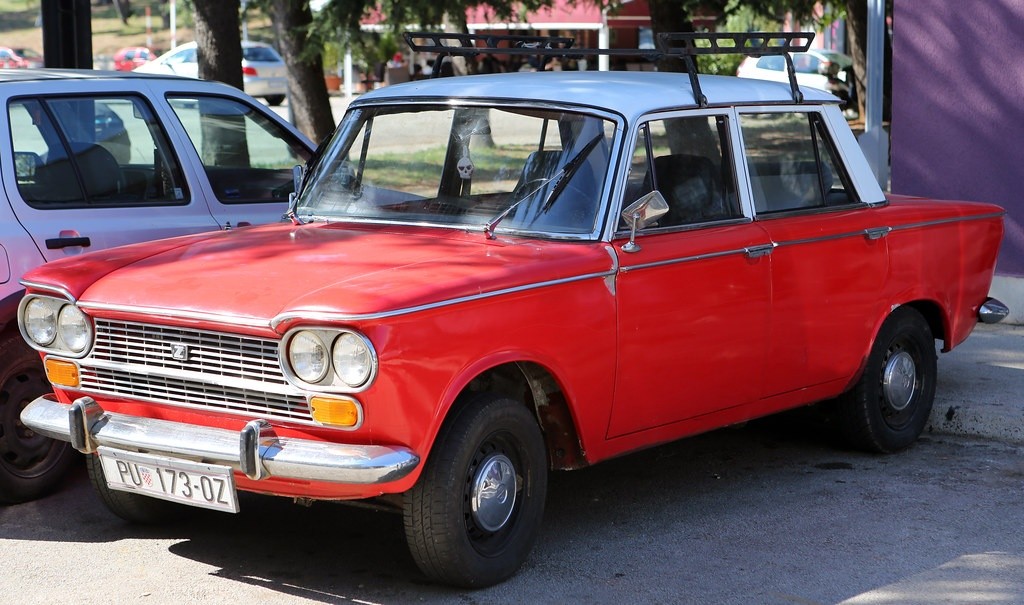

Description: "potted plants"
324 42 341 90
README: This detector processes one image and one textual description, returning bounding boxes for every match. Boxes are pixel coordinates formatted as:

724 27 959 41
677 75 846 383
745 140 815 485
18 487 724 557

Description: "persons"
359 36 576 92
825 64 857 121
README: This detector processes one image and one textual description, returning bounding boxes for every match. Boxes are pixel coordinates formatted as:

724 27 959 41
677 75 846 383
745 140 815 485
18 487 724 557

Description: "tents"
341 0 720 105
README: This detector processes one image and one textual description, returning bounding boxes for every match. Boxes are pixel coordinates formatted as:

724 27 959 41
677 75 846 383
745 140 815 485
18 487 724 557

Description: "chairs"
509 150 597 230
48 144 124 201
637 154 726 228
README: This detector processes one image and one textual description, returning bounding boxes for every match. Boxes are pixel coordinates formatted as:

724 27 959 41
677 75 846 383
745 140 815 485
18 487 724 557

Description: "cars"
0 45 45 69
132 39 288 106
112 47 158 73
0 70 435 503
735 44 864 121
16 29 1011 593
